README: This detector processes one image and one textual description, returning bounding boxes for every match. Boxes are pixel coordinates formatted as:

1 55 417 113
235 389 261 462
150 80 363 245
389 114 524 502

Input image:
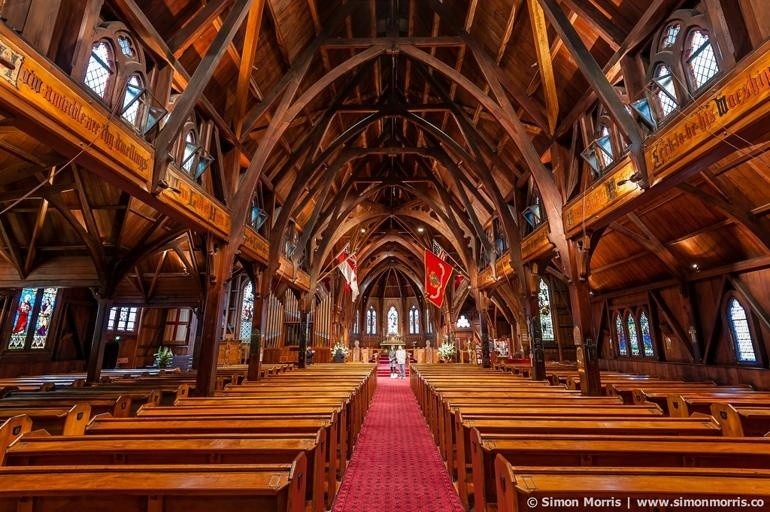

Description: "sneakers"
398 375 405 378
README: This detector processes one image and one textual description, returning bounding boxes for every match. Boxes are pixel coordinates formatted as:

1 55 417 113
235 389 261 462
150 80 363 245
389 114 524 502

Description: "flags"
338 253 361 303
433 241 449 261
424 249 454 309
335 242 351 297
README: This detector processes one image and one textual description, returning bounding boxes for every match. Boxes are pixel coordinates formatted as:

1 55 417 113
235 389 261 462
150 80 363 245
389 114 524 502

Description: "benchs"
0 363 376 511
411 360 770 512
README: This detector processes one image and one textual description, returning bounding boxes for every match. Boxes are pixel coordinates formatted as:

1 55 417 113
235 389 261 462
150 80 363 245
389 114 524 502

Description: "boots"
390 373 399 379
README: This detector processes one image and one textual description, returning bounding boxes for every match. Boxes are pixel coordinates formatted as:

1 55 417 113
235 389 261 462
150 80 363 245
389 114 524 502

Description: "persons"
11 294 32 336
387 345 399 379
395 344 406 379
306 343 316 365
36 298 53 336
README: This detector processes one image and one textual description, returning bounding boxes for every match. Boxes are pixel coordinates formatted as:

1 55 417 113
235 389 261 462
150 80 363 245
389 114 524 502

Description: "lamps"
618 171 647 191
152 178 182 197
